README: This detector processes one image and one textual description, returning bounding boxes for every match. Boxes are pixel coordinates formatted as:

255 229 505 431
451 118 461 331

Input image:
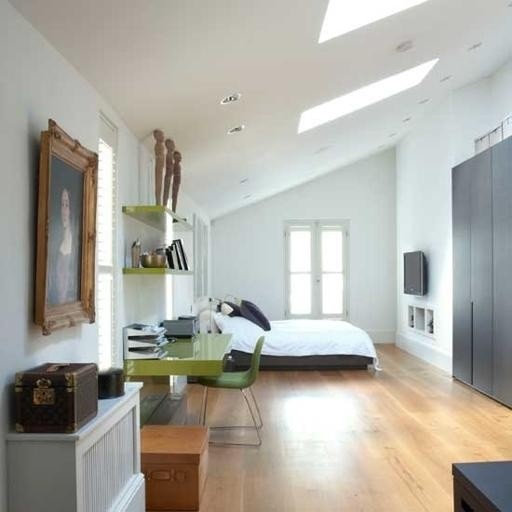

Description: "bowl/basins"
140 254 167 268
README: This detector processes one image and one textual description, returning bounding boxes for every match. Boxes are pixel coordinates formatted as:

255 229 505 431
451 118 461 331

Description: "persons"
163 137 176 206
47 181 80 306
152 128 165 205
172 150 181 213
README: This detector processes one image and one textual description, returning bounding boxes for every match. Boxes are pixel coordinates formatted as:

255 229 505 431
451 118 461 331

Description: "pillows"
215 300 271 332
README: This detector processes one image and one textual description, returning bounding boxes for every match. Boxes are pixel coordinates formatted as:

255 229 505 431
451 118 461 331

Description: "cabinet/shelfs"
6 381 146 512
452 462 512 512
406 305 434 338
122 206 193 275
452 136 512 409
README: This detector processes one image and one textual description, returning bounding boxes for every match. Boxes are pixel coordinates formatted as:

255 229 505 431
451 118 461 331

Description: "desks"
124 334 234 375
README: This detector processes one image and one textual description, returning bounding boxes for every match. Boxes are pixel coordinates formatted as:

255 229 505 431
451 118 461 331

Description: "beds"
192 296 382 371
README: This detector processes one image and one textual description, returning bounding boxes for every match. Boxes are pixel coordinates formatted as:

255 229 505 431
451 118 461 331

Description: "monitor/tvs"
404 251 427 296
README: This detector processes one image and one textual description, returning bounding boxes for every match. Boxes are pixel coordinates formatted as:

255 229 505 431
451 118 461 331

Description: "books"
166 238 189 271
129 324 168 357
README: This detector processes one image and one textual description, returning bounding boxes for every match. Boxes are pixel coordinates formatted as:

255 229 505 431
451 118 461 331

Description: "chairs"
199 335 265 446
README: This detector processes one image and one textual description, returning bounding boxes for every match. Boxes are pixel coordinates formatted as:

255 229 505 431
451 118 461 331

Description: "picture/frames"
34 119 98 335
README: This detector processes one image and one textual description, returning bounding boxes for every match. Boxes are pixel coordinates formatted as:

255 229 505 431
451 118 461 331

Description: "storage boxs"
14 363 98 433
140 425 209 512
